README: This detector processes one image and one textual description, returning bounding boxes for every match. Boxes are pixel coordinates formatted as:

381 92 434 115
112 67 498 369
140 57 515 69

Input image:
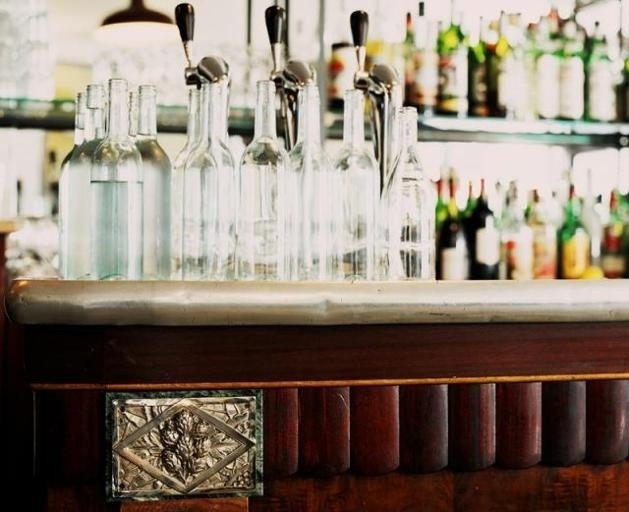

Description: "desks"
0 275 629 512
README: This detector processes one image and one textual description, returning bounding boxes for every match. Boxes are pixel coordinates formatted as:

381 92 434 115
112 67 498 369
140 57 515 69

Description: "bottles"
326 0 629 123
56 77 436 283
435 174 629 280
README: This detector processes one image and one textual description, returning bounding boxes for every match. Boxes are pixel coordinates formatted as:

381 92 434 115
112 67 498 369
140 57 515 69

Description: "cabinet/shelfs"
0 1 628 277
